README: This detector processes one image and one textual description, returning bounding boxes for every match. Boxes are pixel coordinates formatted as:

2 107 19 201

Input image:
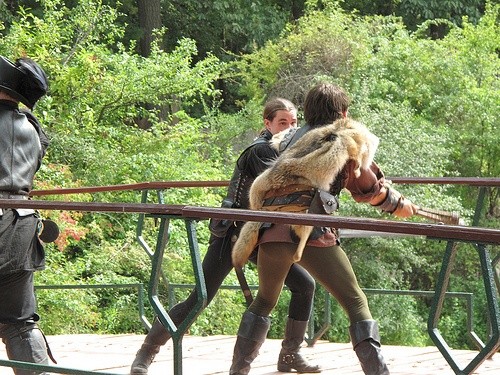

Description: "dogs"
228 118 380 268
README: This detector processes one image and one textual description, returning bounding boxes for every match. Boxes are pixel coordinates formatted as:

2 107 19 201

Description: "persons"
229 83 421 375
0 56 50 375
130 98 322 375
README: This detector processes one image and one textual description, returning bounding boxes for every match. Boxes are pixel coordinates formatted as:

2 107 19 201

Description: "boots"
277 316 322 374
229 308 271 375
130 317 169 375
349 319 390 375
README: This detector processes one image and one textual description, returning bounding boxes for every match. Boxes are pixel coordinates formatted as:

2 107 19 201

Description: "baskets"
262 195 312 206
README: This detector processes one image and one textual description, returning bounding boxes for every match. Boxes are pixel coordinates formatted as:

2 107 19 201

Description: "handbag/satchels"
308 189 338 214
208 200 238 238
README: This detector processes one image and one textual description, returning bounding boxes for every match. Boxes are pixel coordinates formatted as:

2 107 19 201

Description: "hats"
0 55 50 109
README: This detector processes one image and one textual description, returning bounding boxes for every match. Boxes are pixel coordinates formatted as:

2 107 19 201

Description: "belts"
0 190 29 195
